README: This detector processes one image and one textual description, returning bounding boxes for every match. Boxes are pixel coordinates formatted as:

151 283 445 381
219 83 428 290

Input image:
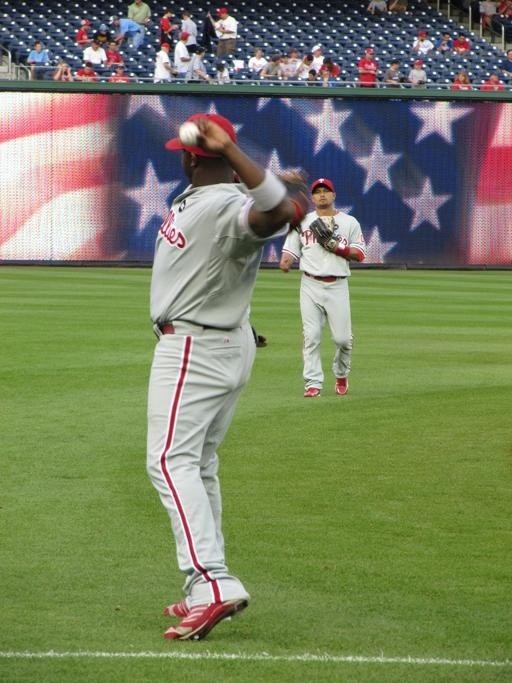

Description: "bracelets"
334 243 350 257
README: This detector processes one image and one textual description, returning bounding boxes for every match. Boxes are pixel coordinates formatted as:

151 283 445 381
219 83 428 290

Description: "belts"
304 271 347 282
159 323 174 334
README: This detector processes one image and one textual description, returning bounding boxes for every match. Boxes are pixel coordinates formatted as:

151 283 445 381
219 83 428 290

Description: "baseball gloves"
310 215 340 253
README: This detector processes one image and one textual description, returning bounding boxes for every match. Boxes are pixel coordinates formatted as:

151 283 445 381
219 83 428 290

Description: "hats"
311 45 321 53
108 15 118 25
310 178 336 195
164 112 238 158
82 19 92 26
415 60 423 64
196 47 206 55
162 43 171 48
94 39 103 46
109 41 118 46
180 32 191 41
216 8 228 16
99 23 110 31
290 48 298 54
365 48 375 55
390 60 401 64
419 31 426 37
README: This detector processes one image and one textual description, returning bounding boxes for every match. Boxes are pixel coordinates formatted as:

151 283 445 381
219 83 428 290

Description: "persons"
280 178 366 397
27 1 512 92
146 113 309 640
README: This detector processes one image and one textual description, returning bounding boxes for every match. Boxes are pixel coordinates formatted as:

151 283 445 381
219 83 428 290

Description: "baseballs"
178 121 198 146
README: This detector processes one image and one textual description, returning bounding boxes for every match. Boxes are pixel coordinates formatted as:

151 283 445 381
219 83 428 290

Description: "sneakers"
304 387 320 397
335 377 348 395
163 598 248 640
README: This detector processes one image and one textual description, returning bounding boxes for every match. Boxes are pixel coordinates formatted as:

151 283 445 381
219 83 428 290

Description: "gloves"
251 326 267 347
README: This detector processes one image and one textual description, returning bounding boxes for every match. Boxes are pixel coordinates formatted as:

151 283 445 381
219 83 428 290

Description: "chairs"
0 0 512 102
